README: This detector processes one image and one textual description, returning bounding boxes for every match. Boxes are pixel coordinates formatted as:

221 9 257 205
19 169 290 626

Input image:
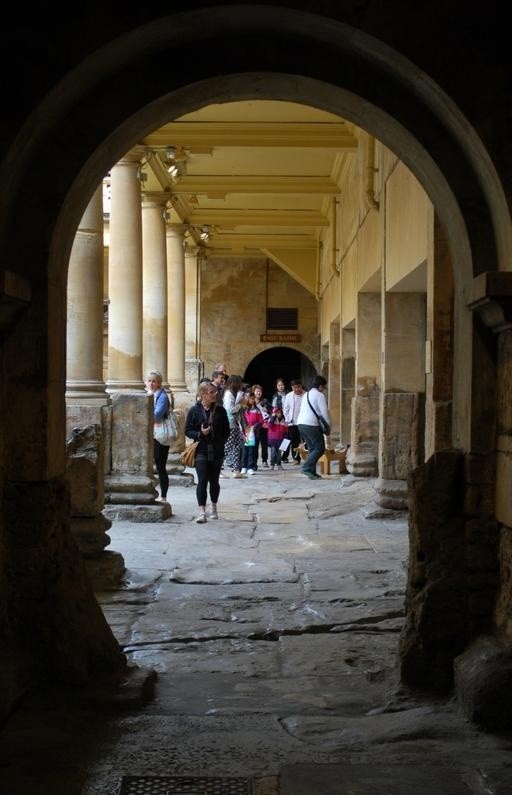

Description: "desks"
294 443 349 475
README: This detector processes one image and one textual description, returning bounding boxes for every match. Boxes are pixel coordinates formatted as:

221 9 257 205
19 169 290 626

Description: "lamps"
163 161 178 175
198 229 209 240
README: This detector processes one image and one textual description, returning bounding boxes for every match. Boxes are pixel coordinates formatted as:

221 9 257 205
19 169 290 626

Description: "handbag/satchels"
318 414 331 436
178 440 199 469
153 406 180 447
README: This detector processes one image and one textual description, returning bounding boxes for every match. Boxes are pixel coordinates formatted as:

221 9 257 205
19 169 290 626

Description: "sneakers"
218 460 324 481
195 513 207 524
209 511 219 520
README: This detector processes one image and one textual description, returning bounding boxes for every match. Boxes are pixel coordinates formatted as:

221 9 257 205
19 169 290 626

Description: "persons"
185 381 230 522
147 371 169 503
200 364 330 479
